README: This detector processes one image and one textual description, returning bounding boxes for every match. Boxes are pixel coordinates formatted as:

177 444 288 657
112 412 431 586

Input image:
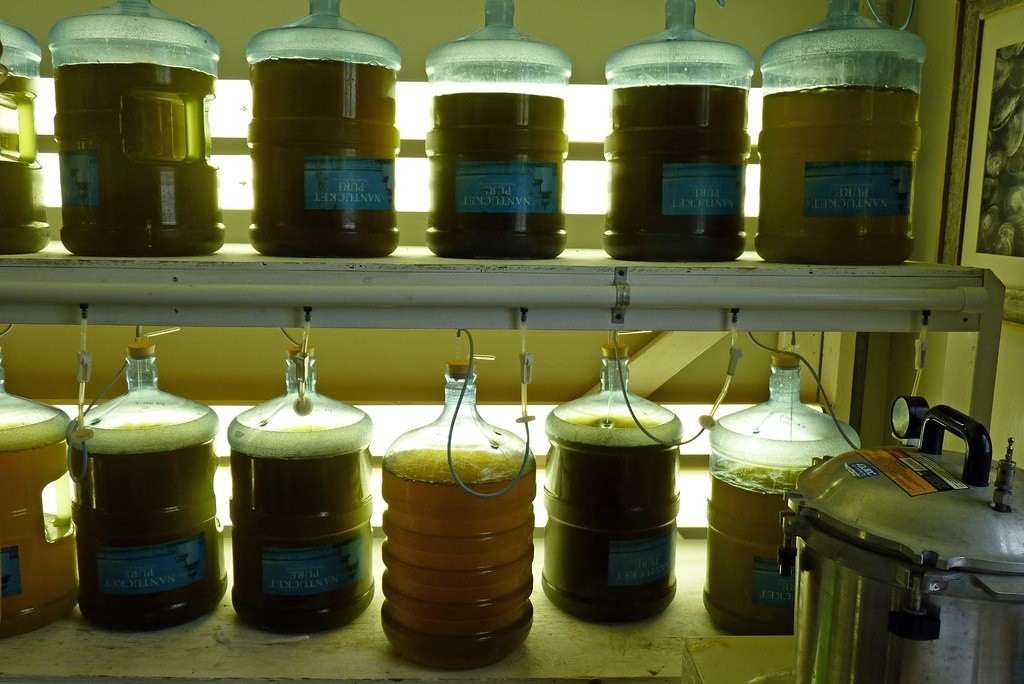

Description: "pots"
778 401 1024 684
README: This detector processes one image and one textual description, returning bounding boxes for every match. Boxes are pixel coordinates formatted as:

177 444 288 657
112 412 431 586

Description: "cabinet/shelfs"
1 252 1005 683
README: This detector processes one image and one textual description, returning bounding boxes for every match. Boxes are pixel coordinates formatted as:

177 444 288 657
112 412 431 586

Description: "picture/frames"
938 1 1024 323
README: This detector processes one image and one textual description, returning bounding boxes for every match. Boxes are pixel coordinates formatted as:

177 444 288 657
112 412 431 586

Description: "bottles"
67 341 226 631
229 348 375 632
423 0 570 258
543 345 681 625
754 2 925 264
0 360 80 643
601 0 754 261
246 0 401 257
48 0 225 255
2 13 43 255
382 364 535 670
703 354 860 637
